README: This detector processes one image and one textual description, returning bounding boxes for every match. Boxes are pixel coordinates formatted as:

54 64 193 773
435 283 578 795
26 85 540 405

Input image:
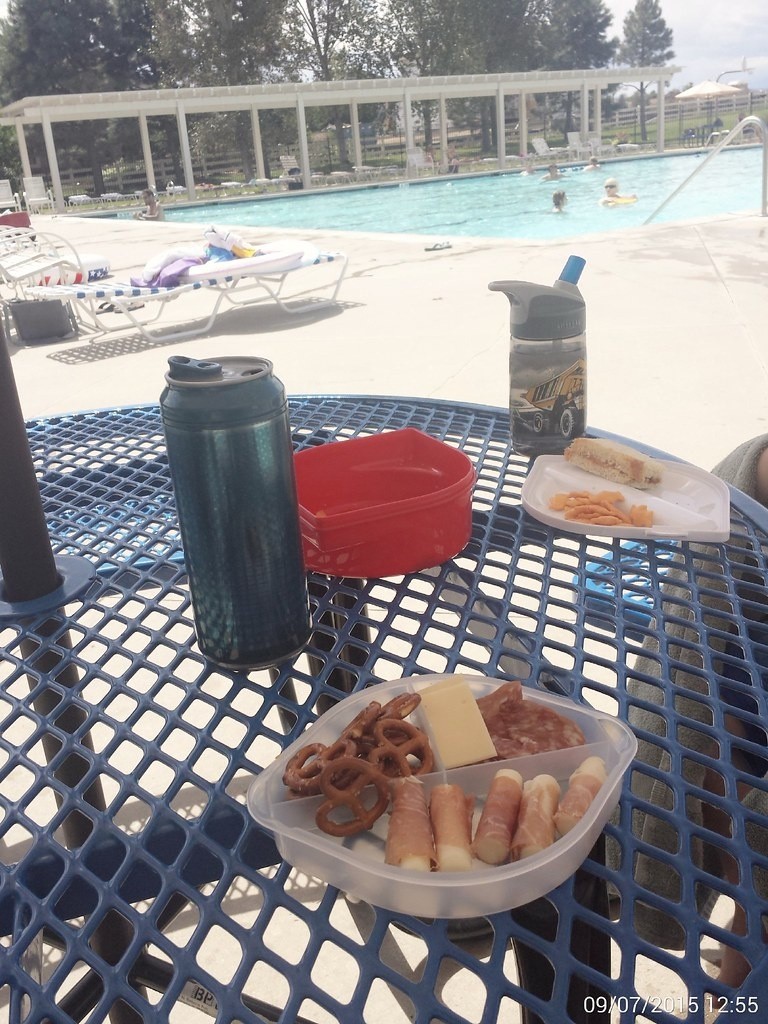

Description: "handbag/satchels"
3 298 79 345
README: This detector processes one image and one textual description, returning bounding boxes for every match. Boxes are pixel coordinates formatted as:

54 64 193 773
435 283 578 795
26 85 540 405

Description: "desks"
0 389 768 1024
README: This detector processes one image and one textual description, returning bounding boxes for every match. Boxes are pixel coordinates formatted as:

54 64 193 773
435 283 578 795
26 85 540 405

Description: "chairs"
280 155 303 183
681 113 755 147
0 179 22 212
67 177 289 210
22 239 350 343
309 165 397 189
0 225 89 326
22 176 55 216
404 147 436 179
442 132 639 181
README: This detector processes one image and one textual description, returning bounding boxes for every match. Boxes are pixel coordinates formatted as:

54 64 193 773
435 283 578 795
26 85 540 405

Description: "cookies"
549 490 654 527
281 691 432 836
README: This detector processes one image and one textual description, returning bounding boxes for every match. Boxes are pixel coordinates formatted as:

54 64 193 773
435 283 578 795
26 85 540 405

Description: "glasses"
604 184 616 189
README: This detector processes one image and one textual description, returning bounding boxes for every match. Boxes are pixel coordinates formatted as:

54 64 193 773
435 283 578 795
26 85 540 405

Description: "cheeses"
413 672 498 771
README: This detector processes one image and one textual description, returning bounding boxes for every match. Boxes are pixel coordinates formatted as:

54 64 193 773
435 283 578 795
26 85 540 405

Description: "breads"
564 438 663 488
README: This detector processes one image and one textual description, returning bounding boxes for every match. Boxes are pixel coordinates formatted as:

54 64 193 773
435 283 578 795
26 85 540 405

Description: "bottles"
487 254 588 457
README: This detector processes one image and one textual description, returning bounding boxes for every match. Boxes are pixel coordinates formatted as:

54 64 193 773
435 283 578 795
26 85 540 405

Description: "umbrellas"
674 79 741 144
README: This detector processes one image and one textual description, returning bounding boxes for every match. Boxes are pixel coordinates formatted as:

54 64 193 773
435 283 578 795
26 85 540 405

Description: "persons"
132 189 164 221
583 156 600 171
520 164 537 176
551 189 569 212
598 177 637 206
540 164 565 180
604 430 768 1023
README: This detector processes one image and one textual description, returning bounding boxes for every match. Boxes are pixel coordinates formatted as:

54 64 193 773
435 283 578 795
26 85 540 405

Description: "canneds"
159 356 312 671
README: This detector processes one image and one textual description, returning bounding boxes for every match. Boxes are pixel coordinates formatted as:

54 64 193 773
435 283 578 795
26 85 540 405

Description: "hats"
604 178 618 185
548 163 557 171
590 156 598 163
552 189 566 203
